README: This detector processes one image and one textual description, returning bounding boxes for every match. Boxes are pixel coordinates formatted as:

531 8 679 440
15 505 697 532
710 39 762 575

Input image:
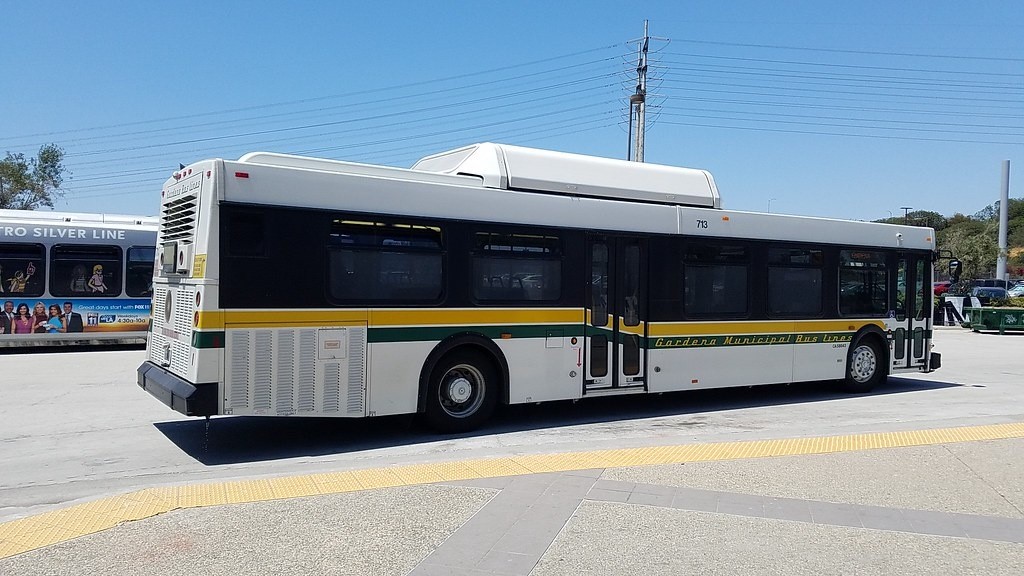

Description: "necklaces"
23 320 26 324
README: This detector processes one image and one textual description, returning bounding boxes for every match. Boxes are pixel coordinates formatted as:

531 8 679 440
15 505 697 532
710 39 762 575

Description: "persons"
0 307 5 333
33 302 48 333
0 301 17 334
11 303 34 333
62 302 83 332
70 275 90 291
47 303 67 333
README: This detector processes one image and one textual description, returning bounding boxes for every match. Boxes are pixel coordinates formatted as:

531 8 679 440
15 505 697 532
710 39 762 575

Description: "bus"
134 139 964 436
0 207 161 354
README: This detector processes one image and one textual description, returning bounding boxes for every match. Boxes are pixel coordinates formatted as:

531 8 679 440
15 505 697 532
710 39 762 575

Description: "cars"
897 276 1024 328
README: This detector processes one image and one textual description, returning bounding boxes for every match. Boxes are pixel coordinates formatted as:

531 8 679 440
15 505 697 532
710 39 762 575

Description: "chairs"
491 277 531 302
